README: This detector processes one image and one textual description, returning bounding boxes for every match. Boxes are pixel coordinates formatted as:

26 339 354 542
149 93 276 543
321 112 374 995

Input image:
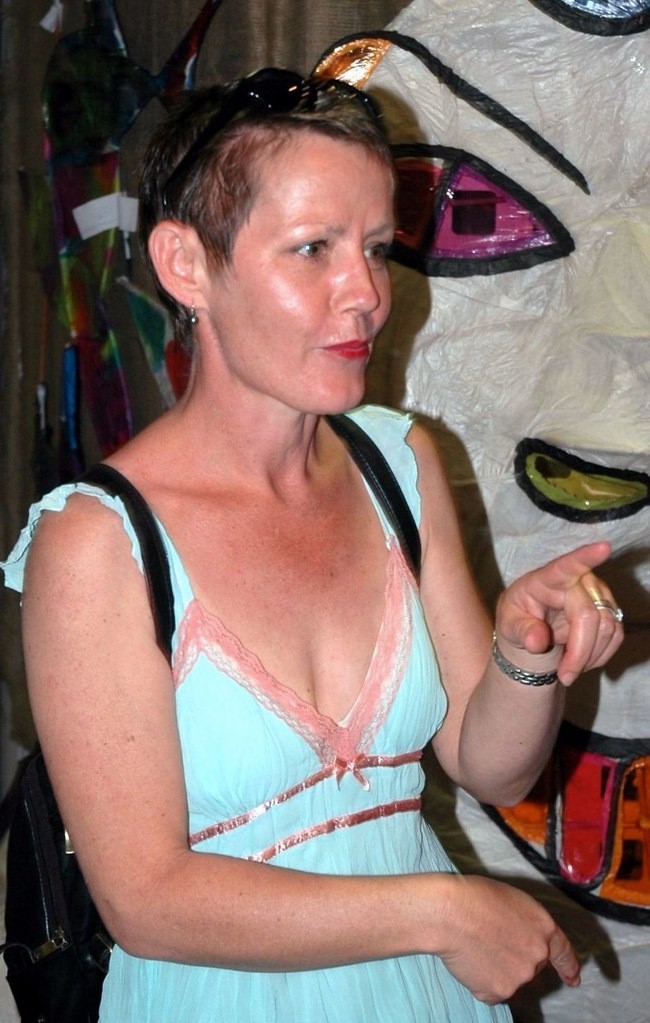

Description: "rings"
594 599 624 622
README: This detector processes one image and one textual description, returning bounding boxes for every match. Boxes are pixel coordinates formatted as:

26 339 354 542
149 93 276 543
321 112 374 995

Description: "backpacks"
0 409 420 1023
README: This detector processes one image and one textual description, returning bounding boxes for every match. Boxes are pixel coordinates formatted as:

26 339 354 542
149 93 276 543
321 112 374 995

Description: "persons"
0 62 624 1023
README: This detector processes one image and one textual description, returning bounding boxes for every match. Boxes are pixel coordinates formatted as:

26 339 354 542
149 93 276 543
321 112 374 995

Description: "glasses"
161 67 381 219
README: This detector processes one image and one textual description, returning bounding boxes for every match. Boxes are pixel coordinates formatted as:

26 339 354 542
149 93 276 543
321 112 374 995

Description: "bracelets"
491 630 557 686
